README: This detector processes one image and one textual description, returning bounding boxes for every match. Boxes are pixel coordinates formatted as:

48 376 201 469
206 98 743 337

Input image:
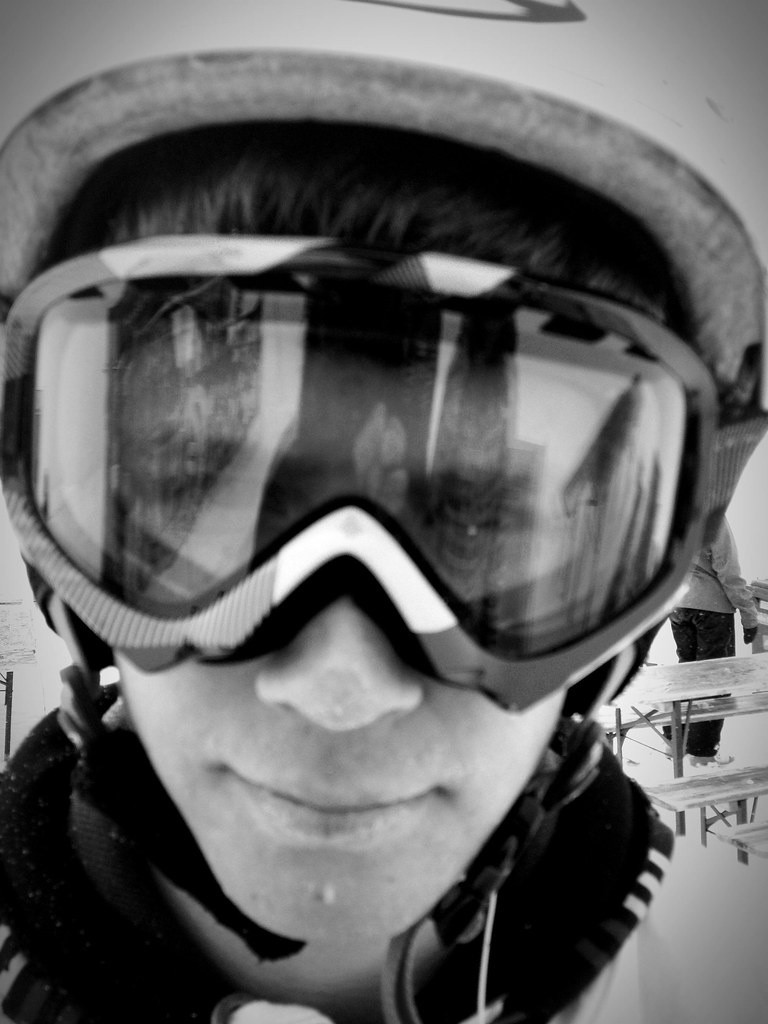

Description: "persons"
662 514 758 764
0 0 768 1024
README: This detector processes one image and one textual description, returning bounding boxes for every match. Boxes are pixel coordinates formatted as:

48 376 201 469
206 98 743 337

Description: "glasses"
0 236 718 711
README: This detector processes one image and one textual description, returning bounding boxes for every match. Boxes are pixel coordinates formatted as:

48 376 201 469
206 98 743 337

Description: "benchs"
596 691 768 762
712 820 768 863
640 763 768 866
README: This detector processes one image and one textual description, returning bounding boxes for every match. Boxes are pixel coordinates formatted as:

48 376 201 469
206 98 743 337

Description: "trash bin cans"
746 578 768 652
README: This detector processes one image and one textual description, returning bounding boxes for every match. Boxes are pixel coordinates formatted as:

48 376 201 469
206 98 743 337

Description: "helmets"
0 0 768 384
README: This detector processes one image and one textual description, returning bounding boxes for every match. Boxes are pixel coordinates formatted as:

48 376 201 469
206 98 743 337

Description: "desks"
605 652 767 836
